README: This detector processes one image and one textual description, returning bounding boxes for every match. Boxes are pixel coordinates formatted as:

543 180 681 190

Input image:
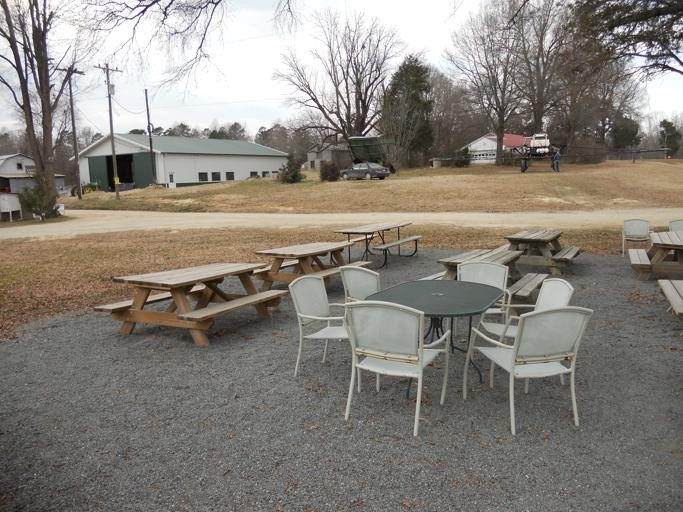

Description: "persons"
519 144 561 172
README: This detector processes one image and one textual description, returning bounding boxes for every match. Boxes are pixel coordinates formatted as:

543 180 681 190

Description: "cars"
339 161 390 182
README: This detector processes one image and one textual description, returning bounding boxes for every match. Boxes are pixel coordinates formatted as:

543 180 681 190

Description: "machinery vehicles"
71 176 105 197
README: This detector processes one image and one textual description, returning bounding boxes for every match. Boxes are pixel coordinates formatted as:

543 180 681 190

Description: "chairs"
339 265 381 303
289 274 362 393
468 278 575 388
463 305 595 436
342 300 451 437
456 261 510 344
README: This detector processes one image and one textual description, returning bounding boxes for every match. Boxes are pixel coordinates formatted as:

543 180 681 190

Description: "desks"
364 280 505 399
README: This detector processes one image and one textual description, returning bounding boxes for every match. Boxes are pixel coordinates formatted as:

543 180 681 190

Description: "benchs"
438 248 549 304
253 242 372 298
92 263 289 348
333 220 423 268
622 219 681 317
492 230 583 276
